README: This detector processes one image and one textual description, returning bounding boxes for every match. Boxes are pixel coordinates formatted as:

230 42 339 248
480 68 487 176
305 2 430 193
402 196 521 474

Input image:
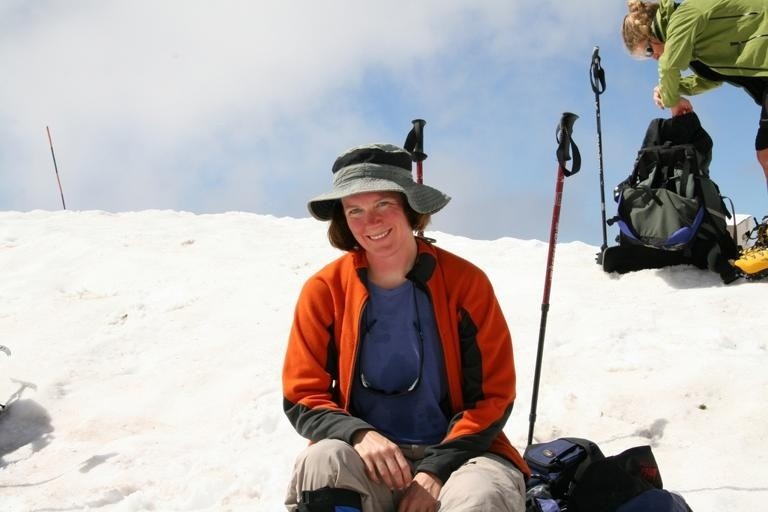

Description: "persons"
281 141 535 511
622 1 768 194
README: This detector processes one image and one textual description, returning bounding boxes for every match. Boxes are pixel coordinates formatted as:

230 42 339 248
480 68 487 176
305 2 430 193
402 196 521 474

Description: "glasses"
645 36 654 58
360 319 424 396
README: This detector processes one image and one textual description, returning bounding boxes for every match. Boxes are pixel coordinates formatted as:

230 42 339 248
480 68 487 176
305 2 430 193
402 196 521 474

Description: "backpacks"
607 141 737 256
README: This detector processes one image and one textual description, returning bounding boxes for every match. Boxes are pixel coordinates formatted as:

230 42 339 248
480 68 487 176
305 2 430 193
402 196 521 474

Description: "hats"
308 144 451 221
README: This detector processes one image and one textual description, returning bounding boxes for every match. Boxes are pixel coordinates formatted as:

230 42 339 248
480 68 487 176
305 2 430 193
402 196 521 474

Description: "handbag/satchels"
524 437 605 497
